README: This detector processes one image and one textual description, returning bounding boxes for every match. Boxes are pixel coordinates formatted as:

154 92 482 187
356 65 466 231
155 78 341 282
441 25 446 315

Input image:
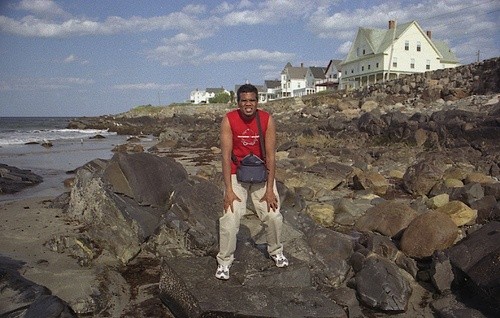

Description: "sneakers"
214 263 230 280
270 251 289 268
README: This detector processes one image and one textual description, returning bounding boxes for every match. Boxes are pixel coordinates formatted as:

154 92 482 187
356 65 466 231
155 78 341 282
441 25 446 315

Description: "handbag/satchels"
236 153 267 184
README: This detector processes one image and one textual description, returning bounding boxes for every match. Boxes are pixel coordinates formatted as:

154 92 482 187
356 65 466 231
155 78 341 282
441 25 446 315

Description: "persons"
215 83 289 280
